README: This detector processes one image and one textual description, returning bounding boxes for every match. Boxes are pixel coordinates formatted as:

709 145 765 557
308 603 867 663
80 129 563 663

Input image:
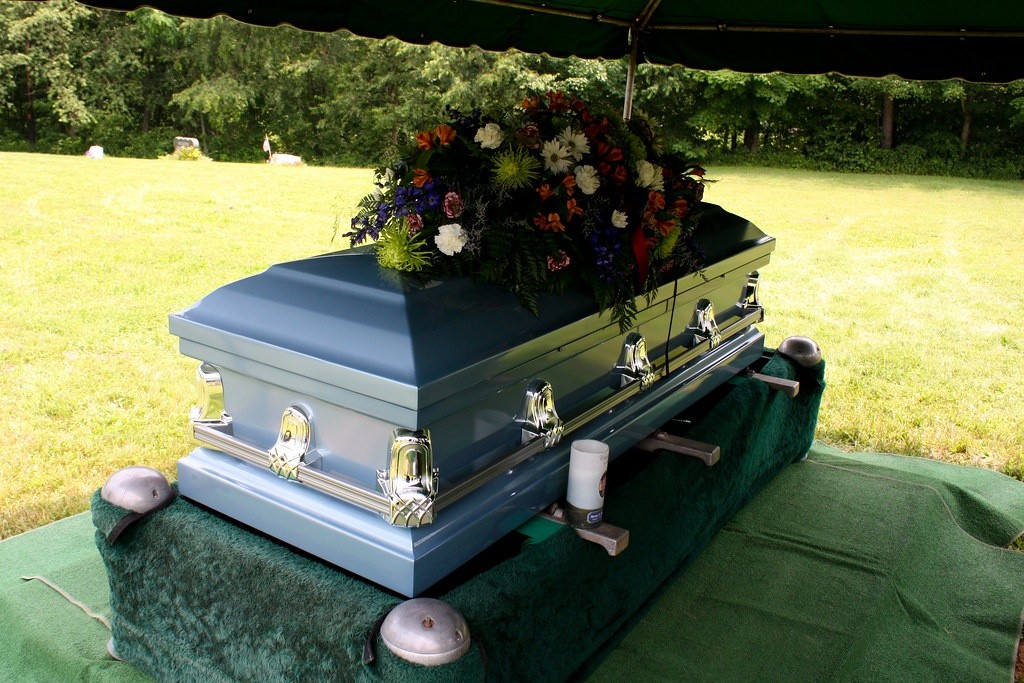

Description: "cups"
564 439 610 529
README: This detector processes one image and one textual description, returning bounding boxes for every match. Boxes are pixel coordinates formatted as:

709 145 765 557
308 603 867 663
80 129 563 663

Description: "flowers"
348 94 707 333
